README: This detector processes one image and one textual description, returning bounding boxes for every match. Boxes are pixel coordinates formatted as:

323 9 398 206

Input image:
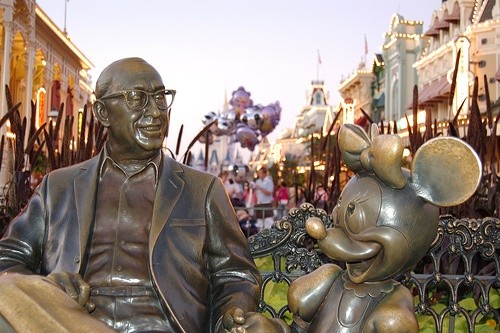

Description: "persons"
0 57 263 333
224 167 289 221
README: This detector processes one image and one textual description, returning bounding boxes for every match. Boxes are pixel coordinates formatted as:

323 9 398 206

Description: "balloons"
198 86 282 152
